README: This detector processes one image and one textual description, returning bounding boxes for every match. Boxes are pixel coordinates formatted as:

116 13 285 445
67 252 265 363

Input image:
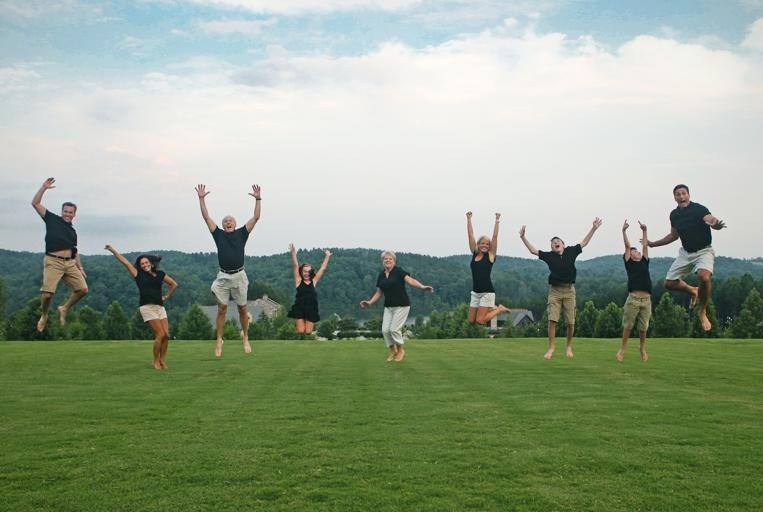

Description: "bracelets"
256 198 262 200
495 220 500 223
78 267 83 270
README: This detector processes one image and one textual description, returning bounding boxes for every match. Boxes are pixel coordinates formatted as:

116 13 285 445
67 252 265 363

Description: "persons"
518 216 602 359
466 211 511 325
359 250 434 362
195 183 261 358
31 178 88 332
639 185 728 331
615 219 653 363
288 243 334 337
104 245 178 369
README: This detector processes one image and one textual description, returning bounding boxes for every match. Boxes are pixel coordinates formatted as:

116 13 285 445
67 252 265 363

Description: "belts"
219 267 244 275
44 251 73 262
